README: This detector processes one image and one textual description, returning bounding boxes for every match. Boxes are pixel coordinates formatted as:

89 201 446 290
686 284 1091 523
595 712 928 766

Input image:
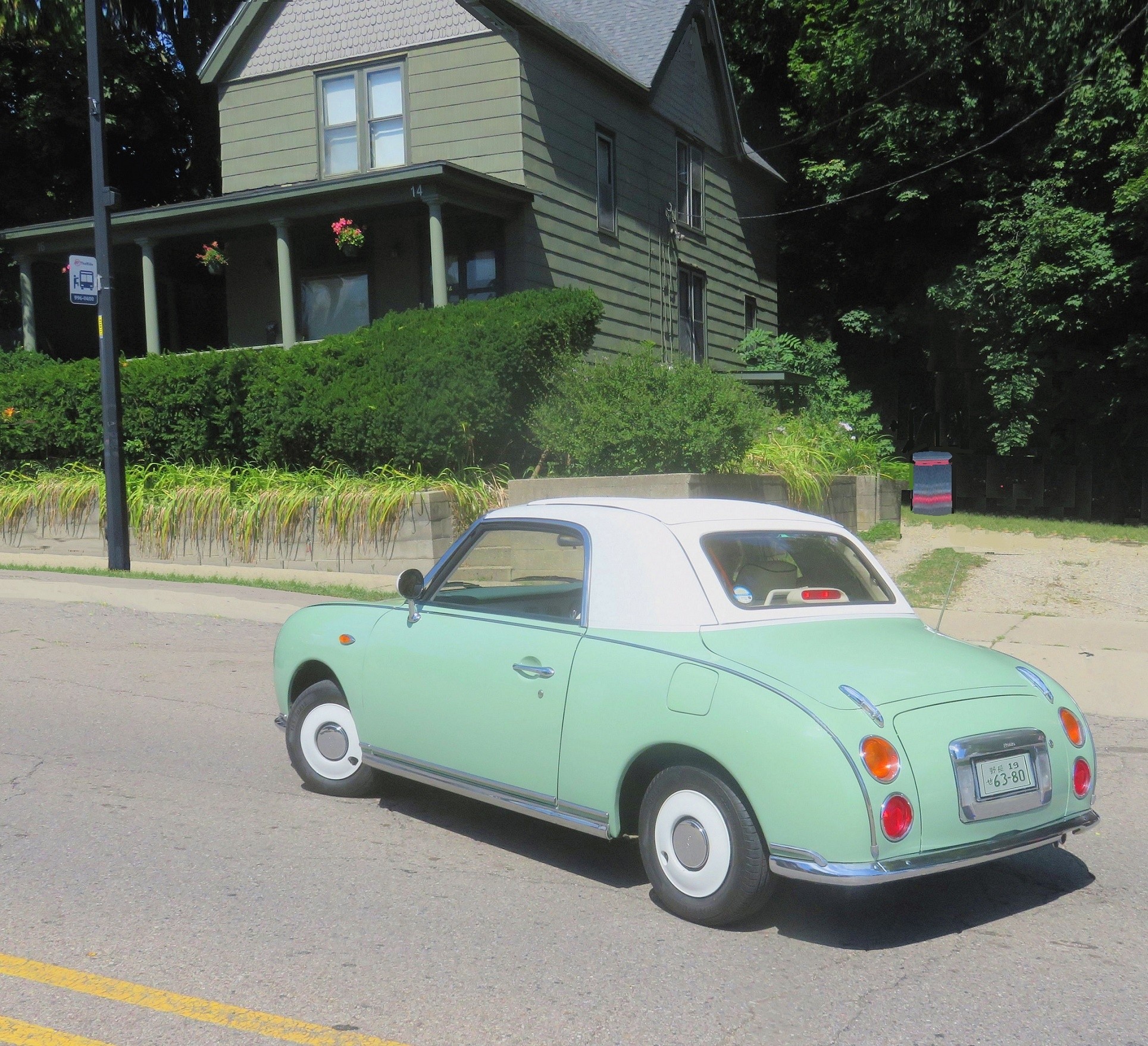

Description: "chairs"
737 560 799 609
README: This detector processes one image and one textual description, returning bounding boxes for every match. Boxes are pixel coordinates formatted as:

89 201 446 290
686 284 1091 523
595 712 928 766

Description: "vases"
207 262 226 276
343 243 364 258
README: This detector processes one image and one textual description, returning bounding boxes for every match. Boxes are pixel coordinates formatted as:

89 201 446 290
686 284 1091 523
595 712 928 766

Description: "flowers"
196 242 230 265
332 218 365 250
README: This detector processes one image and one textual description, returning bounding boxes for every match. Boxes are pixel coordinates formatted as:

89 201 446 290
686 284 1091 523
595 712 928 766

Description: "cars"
271 495 1103 927
